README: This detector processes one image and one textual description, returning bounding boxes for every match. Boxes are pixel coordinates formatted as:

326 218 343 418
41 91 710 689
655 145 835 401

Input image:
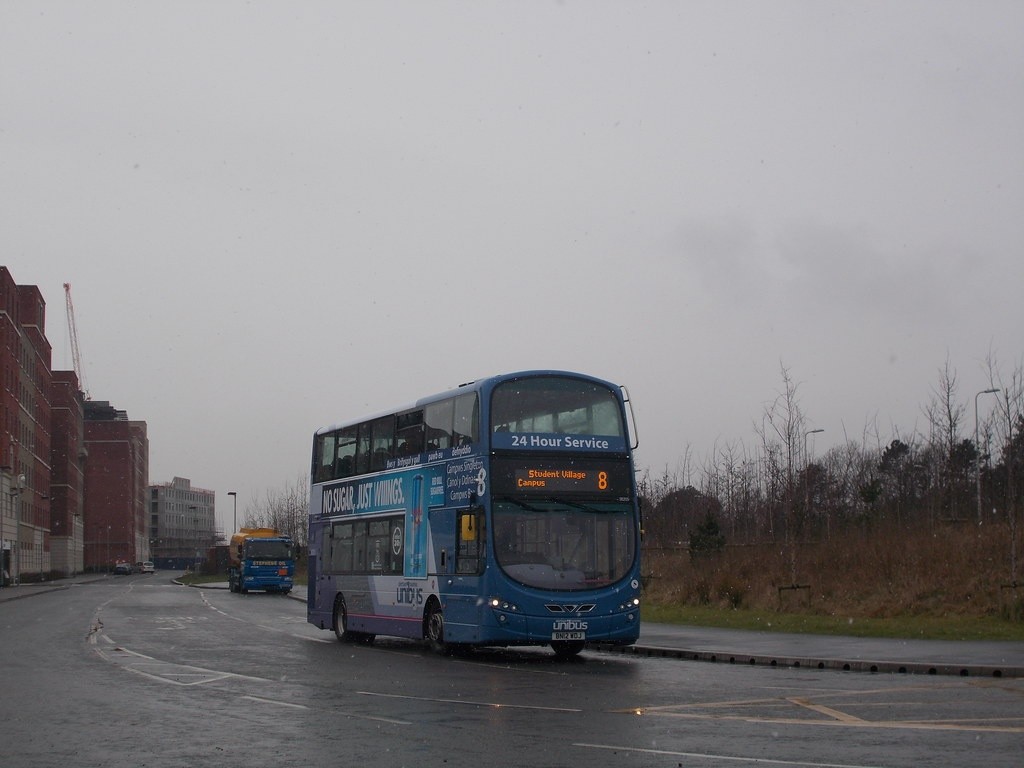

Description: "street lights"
804 429 825 510
974 388 1001 522
40 496 51 582
228 492 236 533
73 513 82 577
189 507 197 562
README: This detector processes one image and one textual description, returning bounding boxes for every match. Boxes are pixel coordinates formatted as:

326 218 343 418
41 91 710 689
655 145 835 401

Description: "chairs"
495 425 513 433
322 435 475 476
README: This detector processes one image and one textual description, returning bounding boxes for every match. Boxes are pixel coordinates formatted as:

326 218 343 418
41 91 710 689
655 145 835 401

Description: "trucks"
226 527 301 596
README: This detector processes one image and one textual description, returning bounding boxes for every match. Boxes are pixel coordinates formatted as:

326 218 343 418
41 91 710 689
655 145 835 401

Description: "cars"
133 561 155 574
113 563 132 575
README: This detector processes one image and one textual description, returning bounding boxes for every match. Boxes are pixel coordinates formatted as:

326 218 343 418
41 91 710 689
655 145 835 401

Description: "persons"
398 432 424 457
553 540 603 574
247 544 255 556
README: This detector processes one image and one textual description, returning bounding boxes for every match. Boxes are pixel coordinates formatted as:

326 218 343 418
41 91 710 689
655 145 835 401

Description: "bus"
307 370 645 659
